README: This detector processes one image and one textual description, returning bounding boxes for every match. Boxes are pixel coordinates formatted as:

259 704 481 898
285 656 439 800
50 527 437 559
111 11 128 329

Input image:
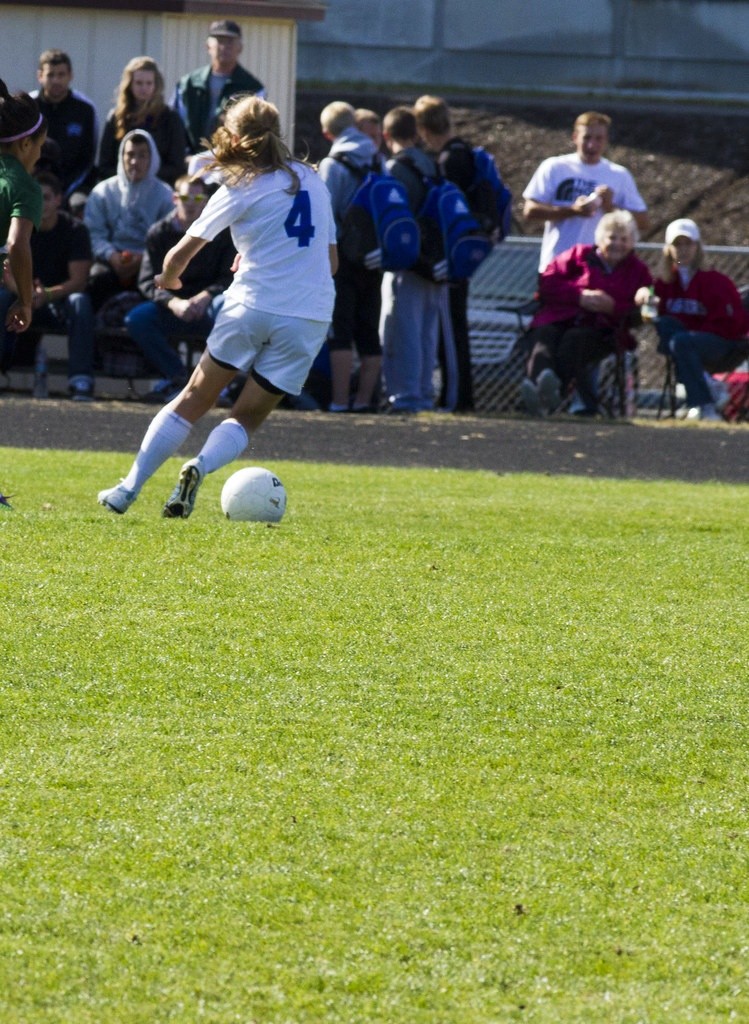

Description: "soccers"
220 467 288 523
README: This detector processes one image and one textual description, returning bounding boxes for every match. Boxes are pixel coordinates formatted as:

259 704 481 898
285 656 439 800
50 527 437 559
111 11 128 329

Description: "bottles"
640 295 658 323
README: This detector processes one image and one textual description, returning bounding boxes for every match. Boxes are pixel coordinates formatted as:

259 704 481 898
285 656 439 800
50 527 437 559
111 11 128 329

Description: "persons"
68 129 175 401
97 94 337 517
523 112 649 274
0 78 48 512
634 218 749 420
123 177 237 403
99 55 194 188
321 96 474 413
31 48 98 209
521 208 654 416
172 19 267 162
0 178 96 400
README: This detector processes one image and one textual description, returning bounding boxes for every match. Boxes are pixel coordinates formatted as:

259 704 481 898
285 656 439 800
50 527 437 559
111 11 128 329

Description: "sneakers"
162 457 205 519
97 484 136 513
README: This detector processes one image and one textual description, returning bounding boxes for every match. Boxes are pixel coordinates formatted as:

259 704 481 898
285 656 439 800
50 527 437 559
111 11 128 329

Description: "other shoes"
521 379 543 415
570 396 585 415
162 368 194 401
537 369 561 409
379 399 405 414
690 403 723 420
214 386 233 408
67 374 97 403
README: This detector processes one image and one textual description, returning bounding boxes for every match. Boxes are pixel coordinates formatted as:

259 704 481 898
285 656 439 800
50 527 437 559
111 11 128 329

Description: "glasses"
176 193 207 205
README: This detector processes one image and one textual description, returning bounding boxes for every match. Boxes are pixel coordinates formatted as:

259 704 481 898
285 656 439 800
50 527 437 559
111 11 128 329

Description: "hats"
209 19 241 39
665 218 700 246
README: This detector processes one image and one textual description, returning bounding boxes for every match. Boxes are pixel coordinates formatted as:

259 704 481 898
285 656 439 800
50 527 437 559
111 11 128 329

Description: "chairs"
656 286 749 422
508 289 642 419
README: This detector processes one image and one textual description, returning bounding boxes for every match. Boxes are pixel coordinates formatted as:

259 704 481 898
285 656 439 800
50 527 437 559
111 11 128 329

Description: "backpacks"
334 152 421 269
435 141 512 242
391 152 492 282
